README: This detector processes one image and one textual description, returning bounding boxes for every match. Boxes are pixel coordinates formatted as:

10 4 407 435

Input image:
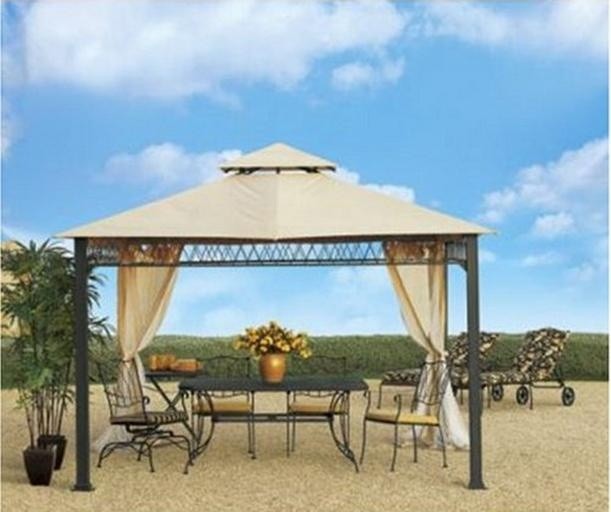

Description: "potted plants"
1 237 120 487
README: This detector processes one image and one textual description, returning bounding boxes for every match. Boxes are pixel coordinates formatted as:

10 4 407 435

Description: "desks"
178 375 373 474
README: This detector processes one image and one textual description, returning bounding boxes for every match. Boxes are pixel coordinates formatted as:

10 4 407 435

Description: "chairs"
359 327 577 473
94 357 194 474
284 354 356 457
193 357 257 458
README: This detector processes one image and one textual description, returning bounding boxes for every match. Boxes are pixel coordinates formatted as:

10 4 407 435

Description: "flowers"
229 318 314 361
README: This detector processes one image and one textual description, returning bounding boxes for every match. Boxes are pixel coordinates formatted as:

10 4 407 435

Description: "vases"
258 351 286 383
